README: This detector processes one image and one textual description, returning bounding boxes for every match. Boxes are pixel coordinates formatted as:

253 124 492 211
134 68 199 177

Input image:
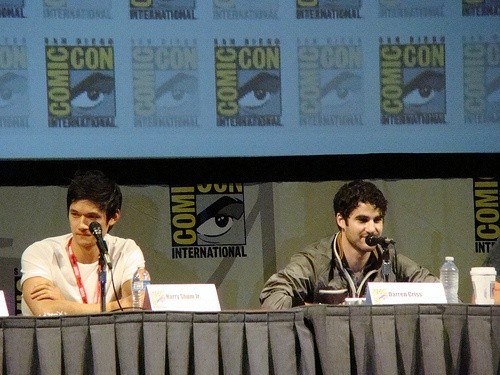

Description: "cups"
470 266 497 306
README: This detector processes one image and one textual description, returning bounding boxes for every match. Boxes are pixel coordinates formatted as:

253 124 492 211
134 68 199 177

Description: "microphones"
365 235 395 246
89 222 112 269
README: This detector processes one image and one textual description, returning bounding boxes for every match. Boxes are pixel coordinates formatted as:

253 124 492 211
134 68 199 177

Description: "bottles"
440 256 459 304
131 260 151 310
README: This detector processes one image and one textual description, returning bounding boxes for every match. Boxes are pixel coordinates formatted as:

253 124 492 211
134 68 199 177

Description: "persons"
260 180 466 309
20 171 145 316
